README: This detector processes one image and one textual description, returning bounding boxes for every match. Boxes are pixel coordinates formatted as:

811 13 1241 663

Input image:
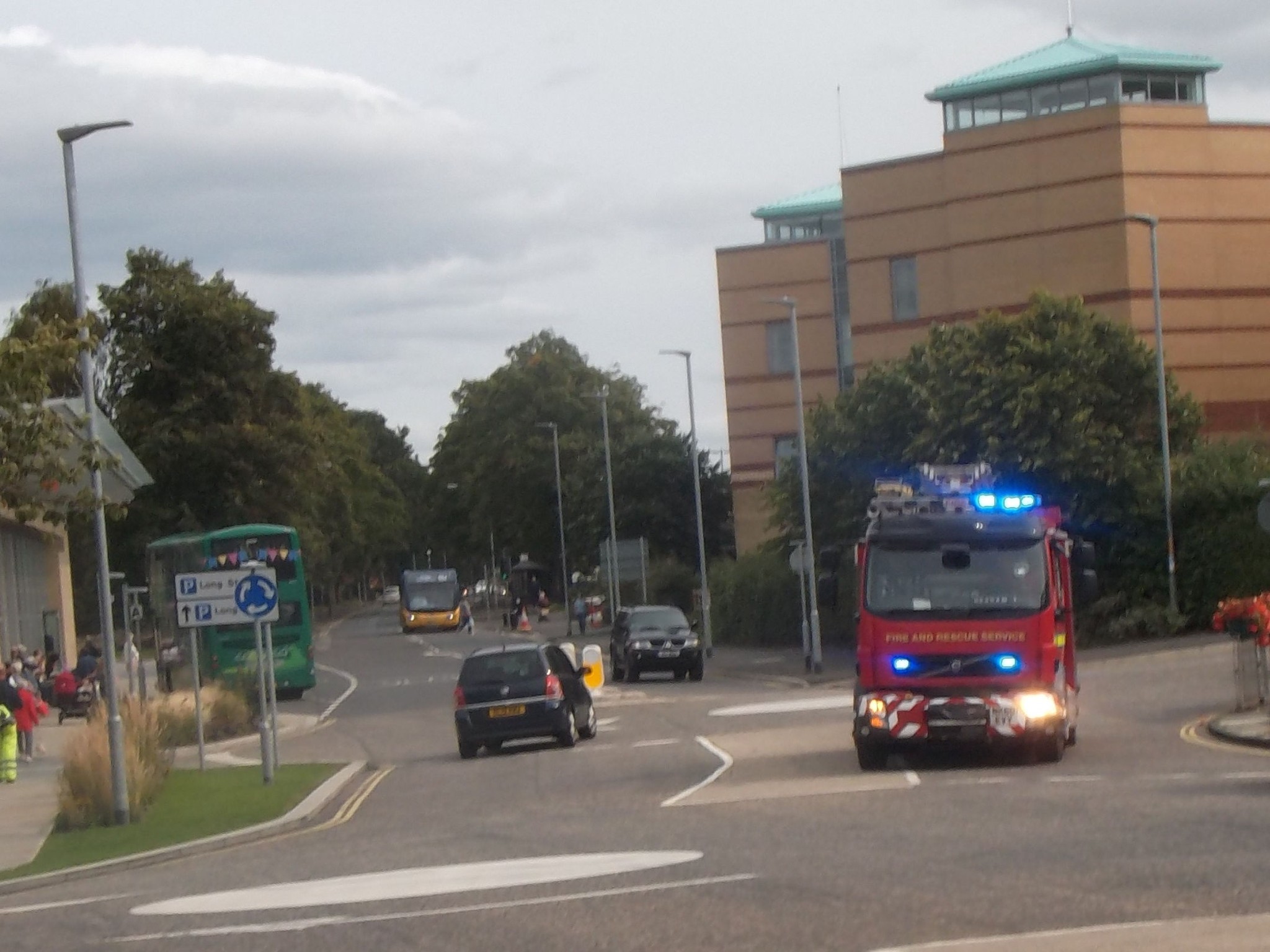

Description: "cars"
382 586 400 606
454 640 596 759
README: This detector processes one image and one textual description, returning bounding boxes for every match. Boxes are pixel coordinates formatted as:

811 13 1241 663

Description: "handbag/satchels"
469 618 474 626
541 609 550 616
35 700 48 716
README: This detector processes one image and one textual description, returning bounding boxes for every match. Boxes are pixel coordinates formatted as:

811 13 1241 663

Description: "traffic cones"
517 606 532 631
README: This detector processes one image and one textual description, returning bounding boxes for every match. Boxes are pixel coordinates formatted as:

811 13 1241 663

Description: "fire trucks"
852 460 1079 772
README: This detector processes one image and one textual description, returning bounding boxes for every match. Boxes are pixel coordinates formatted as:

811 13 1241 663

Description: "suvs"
610 603 703 683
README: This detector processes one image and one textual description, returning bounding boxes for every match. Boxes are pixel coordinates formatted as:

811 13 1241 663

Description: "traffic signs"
175 595 280 630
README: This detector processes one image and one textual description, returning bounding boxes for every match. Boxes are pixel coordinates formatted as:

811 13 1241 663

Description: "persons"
570 591 590 637
537 591 551 622
509 597 524 631
122 633 140 693
457 596 474 635
76 634 104 677
0 642 51 785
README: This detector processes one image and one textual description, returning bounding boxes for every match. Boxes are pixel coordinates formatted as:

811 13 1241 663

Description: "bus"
145 522 315 705
399 568 461 635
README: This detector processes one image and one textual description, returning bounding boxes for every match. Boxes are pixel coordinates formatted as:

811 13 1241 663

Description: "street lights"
58 118 137 828
579 392 621 616
657 347 713 660
533 420 573 636
1127 211 1179 615
761 295 824 678
95 570 126 688
120 584 149 696
